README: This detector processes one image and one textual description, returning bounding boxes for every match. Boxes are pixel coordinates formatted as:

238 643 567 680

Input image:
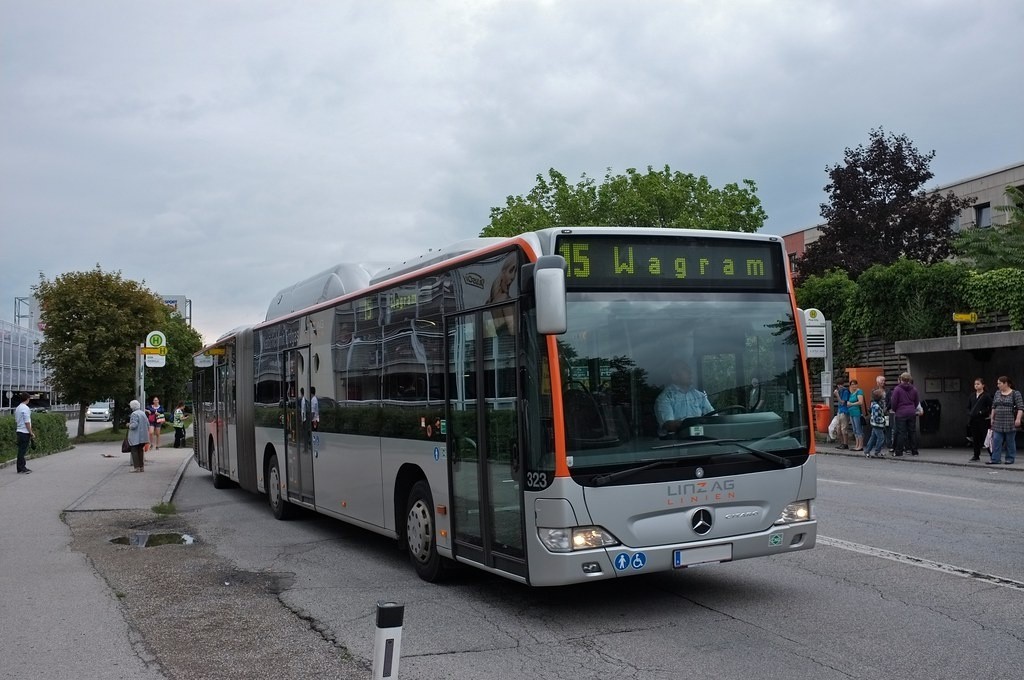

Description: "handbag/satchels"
984 425 995 453
860 413 870 425
1012 391 1024 428
122 430 132 453
157 414 165 423
828 416 841 439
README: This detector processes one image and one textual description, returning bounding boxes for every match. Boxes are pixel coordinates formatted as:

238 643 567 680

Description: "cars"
86 402 113 422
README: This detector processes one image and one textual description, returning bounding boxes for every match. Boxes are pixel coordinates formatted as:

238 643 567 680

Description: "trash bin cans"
919 399 942 435
813 404 830 433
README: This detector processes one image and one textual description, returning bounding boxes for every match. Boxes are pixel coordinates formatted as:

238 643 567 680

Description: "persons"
300 387 319 453
145 397 164 450
14 392 35 474
834 373 923 459
653 359 712 437
968 376 1024 464
170 400 189 448
126 400 151 472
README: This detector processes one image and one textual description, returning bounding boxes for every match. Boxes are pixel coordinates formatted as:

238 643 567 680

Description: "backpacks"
169 412 174 423
305 398 311 416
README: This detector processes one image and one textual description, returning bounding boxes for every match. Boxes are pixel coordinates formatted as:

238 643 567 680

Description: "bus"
190 226 818 586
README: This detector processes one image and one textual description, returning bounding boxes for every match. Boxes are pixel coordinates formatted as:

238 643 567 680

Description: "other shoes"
906 450 911 455
889 448 894 452
850 447 863 451
129 467 140 472
985 460 1002 464
140 467 144 472
892 452 903 456
17 469 32 474
864 452 870 459
912 450 918 456
969 456 980 462
870 452 884 459
1005 460 1014 464
835 444 849 449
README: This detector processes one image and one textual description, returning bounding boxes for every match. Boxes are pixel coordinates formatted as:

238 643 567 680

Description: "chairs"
562 378 619 452
